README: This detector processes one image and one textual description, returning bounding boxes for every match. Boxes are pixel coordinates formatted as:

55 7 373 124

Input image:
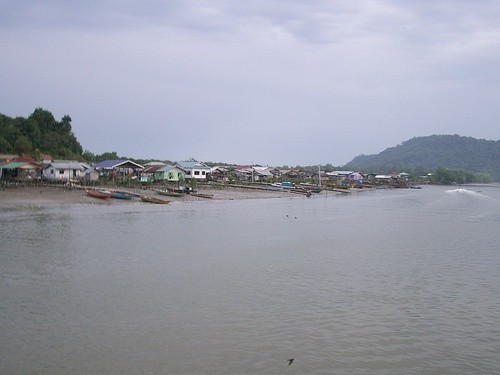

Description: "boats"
84 186 216 204
271 181 423 197
